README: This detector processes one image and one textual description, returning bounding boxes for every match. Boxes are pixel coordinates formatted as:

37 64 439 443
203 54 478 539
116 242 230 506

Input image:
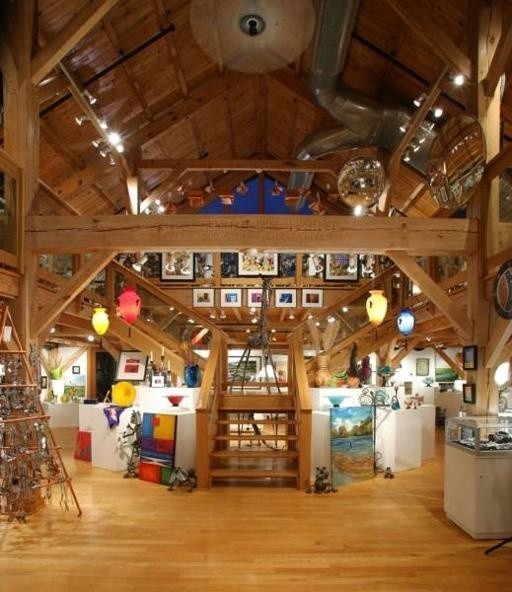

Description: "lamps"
119 253 141 324
91 269 110 335
396 277 415 337
366 254 388 325
57 59 118 165
399 62 461 162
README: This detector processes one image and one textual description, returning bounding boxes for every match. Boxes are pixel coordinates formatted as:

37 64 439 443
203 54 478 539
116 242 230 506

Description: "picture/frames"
324 253 359 282
237 252 280 277
115 350 147 381
152 376 165 387
160 252 194 282
463 345 477 404
192 289 324 308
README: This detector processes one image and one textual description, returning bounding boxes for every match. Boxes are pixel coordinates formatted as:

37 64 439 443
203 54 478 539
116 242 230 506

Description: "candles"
151 351 153 360
162 346 164 355
167 360 171 370
